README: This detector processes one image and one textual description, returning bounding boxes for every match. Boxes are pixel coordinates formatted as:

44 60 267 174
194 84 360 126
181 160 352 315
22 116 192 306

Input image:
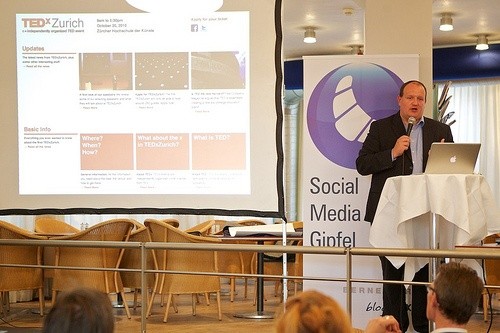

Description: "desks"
206 235 302 319
369 174 500 333
35 233 142 308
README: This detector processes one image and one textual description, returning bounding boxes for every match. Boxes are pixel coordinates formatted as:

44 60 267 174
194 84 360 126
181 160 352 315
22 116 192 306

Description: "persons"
41 289 115 333
363 262 484 333
355 81 454 333
274 291 353 333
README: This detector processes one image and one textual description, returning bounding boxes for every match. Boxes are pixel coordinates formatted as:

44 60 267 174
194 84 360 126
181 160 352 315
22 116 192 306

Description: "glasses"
426 284 436 293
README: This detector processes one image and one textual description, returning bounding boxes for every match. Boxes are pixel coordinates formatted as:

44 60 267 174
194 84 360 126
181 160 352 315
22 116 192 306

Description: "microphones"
406 117 416 137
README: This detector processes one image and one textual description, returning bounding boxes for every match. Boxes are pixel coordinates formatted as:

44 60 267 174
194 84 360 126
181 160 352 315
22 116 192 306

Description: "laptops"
421 142 481 174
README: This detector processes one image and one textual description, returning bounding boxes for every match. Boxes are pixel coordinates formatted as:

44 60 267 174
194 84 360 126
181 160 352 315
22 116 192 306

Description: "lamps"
476 34 489 50
303 28 316 43
439 13 454 32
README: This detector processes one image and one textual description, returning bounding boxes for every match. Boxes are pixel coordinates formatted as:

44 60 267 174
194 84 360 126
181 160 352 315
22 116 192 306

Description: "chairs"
481 232 500 322
0 217 305 323
